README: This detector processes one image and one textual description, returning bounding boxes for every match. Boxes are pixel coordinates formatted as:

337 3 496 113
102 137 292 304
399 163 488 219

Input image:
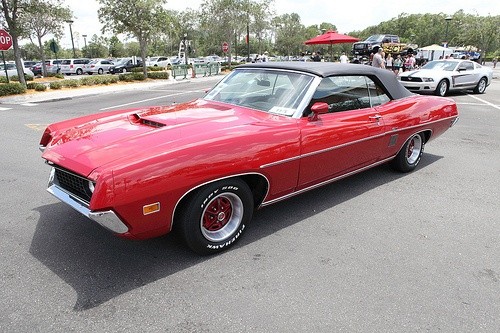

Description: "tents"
418 43 451 61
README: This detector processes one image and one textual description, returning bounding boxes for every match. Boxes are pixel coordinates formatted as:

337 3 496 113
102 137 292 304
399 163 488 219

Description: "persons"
340 47 426 78
439 53 470 60
492 56 497 68
301 51 321 62
477 56 482 64
255 55 260 63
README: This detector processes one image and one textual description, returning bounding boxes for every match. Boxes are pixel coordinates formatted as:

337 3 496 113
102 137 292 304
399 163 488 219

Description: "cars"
231 53 313 64
398 58 494 97
39 62 461 255
0 54 228 79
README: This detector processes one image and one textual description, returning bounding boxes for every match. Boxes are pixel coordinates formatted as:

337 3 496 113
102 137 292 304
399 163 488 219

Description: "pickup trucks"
351 33 419 59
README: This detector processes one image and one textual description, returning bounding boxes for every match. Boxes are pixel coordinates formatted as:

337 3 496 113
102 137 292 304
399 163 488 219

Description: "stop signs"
222 42 229 53
0 29 13 51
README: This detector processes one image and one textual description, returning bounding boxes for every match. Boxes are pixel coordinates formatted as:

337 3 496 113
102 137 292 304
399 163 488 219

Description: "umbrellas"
453 45 481 51
304 27 361 62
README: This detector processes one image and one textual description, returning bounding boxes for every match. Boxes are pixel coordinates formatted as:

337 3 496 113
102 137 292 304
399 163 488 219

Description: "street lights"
444 17 454 42
66 19 76 58
82 34 87 48
